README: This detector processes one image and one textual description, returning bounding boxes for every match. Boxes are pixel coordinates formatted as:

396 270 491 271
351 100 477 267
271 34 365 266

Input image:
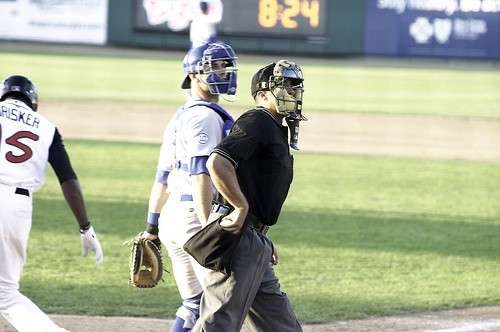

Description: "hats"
251 62 304 97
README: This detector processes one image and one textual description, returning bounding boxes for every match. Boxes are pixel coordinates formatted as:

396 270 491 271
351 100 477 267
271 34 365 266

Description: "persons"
137 40 240 332
0 74 103 332
185 60 308 332
190 1 212 54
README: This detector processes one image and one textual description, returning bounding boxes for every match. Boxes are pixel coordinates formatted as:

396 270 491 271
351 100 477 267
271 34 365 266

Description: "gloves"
78 222 104 263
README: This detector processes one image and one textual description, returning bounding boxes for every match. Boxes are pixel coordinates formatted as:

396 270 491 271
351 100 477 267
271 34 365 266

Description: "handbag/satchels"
182 214 246 277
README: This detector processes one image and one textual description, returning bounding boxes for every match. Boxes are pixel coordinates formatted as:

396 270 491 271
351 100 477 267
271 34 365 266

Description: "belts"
15 187 28 196
181 194 193 201
210 204 268 234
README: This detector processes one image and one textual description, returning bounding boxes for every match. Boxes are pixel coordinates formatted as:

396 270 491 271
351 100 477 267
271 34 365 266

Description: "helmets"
181 40 238 95
0 75 38 112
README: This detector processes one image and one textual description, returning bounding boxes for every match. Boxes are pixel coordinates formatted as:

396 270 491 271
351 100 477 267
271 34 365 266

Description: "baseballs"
220 219 233 227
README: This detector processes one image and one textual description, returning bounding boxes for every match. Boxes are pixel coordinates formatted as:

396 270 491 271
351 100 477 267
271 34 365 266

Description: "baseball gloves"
122 232 171 288
